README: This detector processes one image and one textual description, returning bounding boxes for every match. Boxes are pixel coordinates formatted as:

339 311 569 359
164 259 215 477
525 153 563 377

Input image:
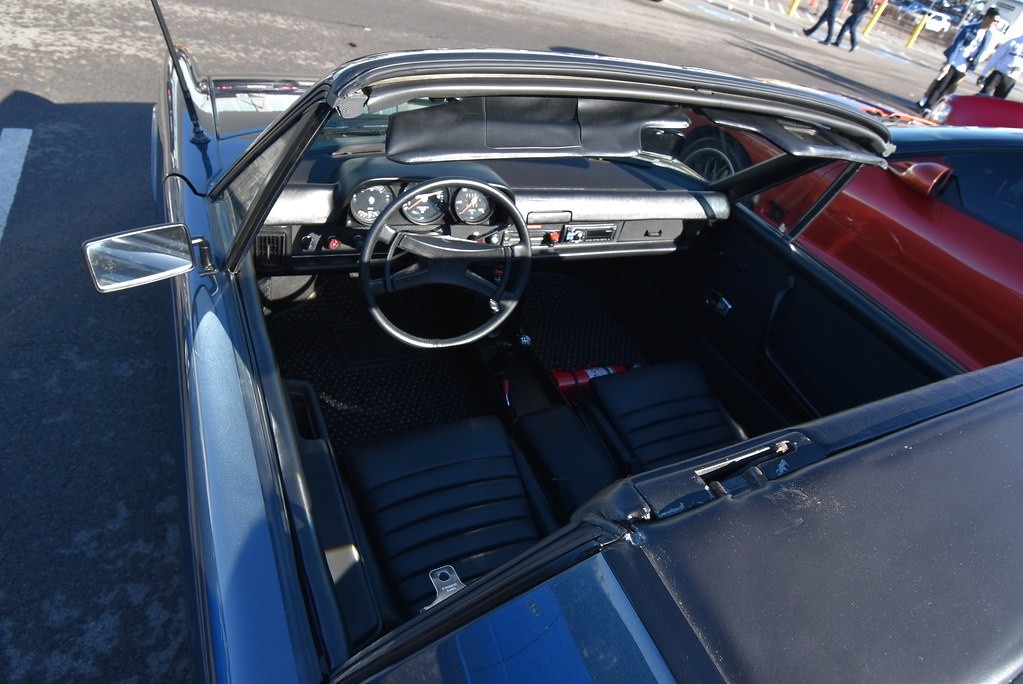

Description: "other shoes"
831 41 840 47
818 40 829 45
803 27 810 36
849 43 857 52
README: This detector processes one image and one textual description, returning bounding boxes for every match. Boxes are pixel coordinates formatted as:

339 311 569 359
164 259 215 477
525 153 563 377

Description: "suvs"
902 8 952 38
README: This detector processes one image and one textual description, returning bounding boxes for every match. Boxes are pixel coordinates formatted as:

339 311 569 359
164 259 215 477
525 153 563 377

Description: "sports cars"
86 43 1023 681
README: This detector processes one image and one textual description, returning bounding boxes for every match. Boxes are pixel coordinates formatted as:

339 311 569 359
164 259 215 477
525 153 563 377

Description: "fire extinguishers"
549 365 629 404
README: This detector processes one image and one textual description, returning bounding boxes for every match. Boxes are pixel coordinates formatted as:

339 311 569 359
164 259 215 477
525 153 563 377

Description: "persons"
976 36 1023 99
916 7 1002 115
831 0 875 51
803 0 844 45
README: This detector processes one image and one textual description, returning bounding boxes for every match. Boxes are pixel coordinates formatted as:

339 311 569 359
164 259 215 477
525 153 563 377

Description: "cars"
635 92 1023 378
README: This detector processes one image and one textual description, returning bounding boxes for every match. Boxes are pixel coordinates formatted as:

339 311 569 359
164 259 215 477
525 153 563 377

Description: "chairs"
343 358 743 617
